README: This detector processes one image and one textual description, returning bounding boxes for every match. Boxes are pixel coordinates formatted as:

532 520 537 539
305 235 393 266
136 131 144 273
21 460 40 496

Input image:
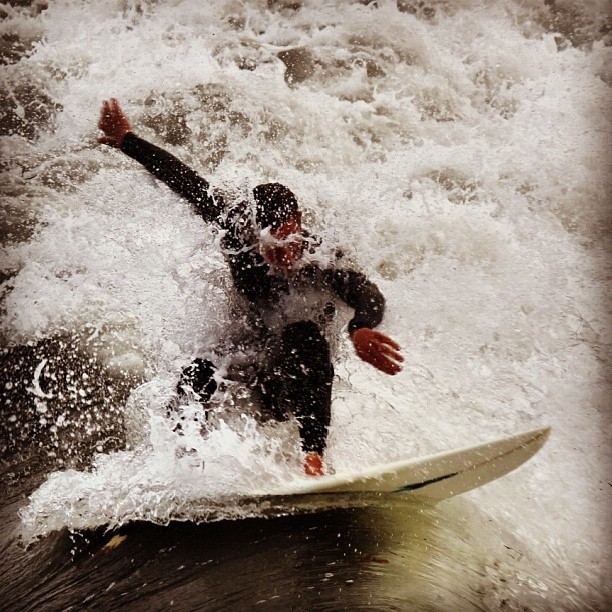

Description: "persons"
96 96 405 479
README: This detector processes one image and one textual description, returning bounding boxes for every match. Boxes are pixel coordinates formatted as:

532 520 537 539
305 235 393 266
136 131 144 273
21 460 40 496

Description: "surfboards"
259 425 552 502
